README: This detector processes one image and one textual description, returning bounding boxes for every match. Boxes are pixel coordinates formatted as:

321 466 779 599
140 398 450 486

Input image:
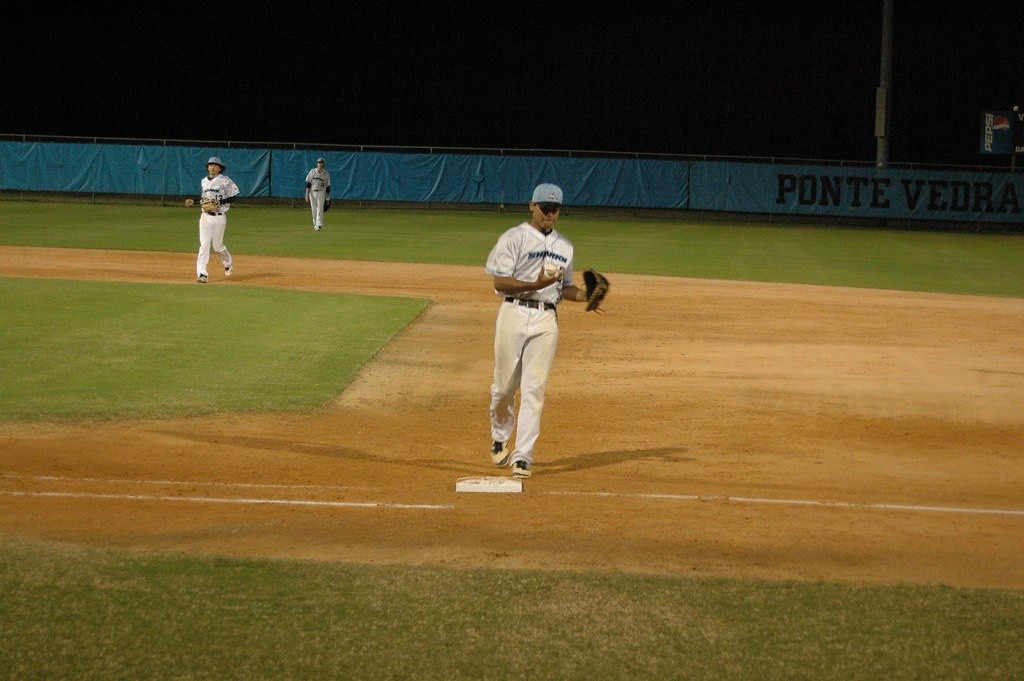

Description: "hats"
532 183 564 204
204 157 225 166
315 158 324 163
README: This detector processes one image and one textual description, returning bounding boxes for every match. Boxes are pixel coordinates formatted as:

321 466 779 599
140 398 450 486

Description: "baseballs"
545 264 557 275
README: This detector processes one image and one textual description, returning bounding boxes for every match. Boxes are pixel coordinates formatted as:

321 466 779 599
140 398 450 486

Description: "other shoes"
313 226 322 232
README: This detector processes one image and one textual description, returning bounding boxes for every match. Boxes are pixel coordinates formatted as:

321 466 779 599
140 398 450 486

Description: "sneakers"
224 260 234 277
490 439 510 468
196 274 208 282
512 461 532 479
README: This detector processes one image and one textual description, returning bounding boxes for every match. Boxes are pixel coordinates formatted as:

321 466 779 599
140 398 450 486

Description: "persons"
485 183 610 478
185 157 241 283
305 157 331 232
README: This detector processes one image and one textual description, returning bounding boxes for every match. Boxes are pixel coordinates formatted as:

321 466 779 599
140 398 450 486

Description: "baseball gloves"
200 197 220 212
324 200 331 212
582 268 610 313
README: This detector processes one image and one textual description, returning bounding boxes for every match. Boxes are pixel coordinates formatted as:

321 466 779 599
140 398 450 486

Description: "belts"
314 190 319 192
505 296 555 311
208 212 223 216
